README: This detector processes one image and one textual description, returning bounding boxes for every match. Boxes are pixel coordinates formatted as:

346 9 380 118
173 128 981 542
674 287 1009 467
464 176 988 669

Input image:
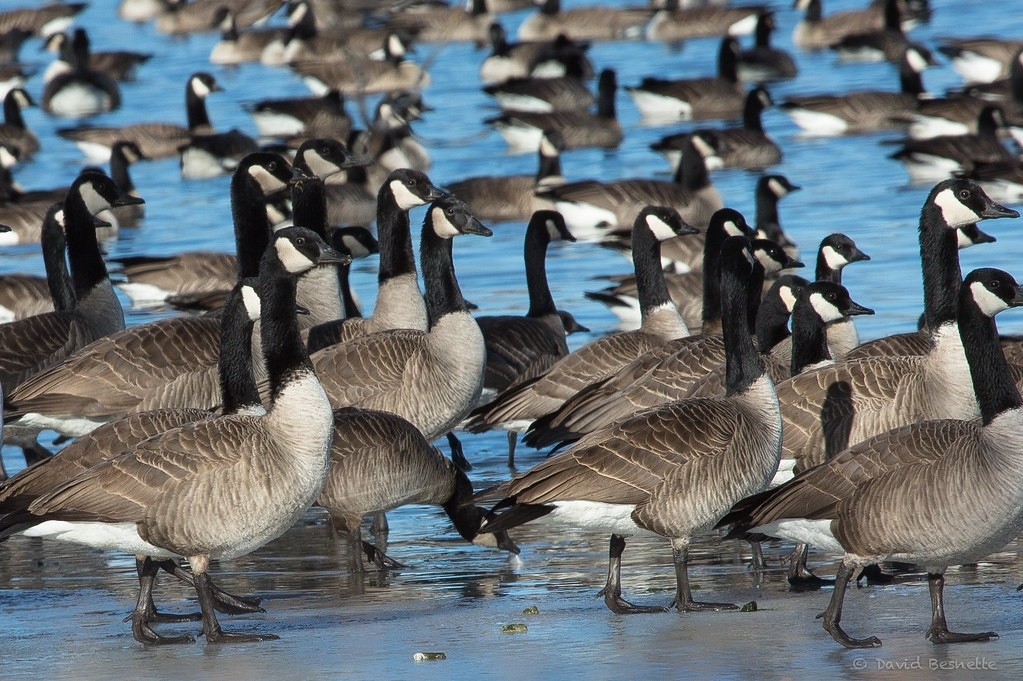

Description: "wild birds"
0 0 1023 648
